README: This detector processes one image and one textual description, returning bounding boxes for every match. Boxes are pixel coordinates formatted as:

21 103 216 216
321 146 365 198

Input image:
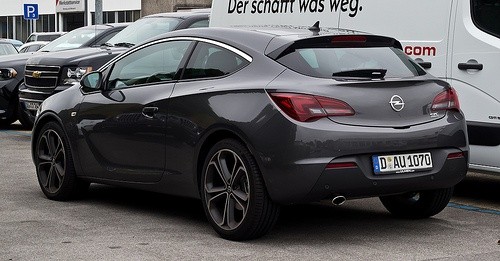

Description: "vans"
209 0 499 176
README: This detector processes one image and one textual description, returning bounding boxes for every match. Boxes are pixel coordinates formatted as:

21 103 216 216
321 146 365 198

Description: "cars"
0 30 70 59
30 20 470 240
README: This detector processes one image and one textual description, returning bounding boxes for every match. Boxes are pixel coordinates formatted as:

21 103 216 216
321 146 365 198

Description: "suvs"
0 24 136 125
18 12 210 116
